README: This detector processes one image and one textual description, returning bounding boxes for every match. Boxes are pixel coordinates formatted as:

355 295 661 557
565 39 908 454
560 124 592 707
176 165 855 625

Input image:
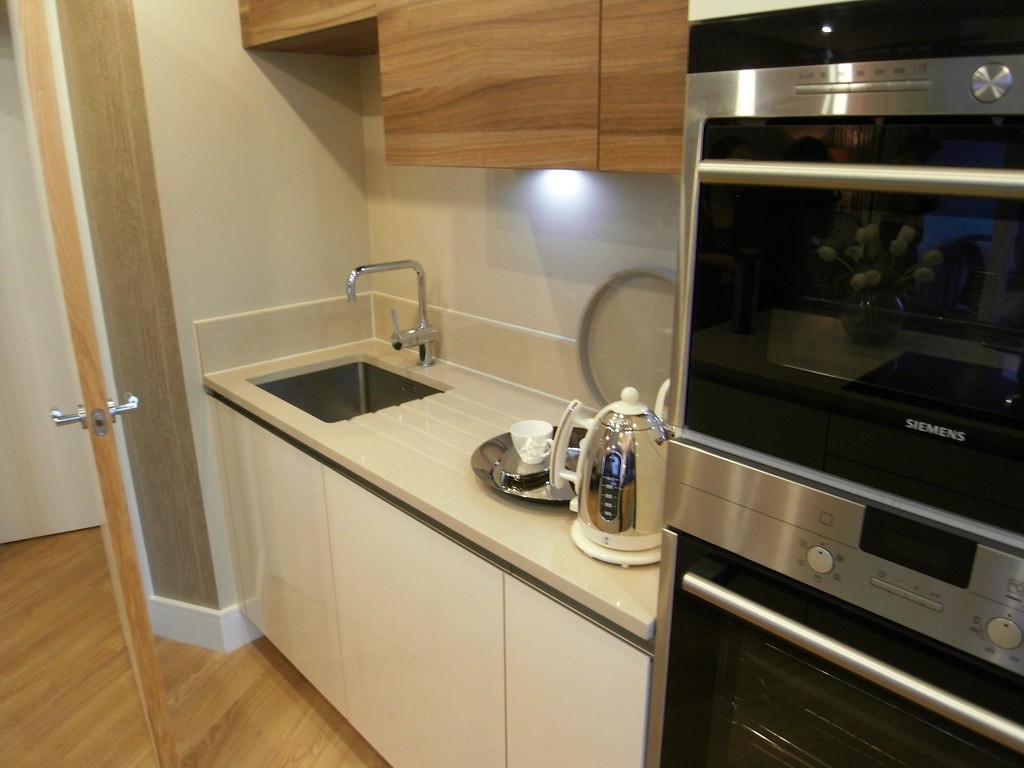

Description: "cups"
510 420 554 465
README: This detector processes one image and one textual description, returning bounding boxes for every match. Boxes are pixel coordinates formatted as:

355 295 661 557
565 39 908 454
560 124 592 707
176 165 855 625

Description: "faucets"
980 340 1024 386
347 259 436 366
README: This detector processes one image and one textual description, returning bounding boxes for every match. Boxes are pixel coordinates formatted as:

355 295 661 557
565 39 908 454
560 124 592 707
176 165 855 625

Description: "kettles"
550 378 671 569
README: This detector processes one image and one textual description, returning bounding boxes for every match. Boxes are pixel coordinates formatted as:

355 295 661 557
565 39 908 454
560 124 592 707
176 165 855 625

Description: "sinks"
245 354 454 427
840 345 1023 435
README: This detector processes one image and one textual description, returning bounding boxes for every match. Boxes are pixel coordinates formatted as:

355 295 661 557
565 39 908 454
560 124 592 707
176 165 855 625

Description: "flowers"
816 223 944 294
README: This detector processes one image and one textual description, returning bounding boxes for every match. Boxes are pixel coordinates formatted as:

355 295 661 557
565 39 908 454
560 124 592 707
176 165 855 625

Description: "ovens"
645 0 1024 767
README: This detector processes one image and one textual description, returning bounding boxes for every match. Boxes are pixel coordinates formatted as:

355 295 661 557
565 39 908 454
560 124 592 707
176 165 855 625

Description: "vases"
842 293 905 342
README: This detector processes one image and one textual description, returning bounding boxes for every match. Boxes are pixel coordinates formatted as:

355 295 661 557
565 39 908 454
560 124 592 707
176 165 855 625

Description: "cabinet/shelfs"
377 0 688 172
204 391 652 768
239 0 379 56
685 377 1023 540
